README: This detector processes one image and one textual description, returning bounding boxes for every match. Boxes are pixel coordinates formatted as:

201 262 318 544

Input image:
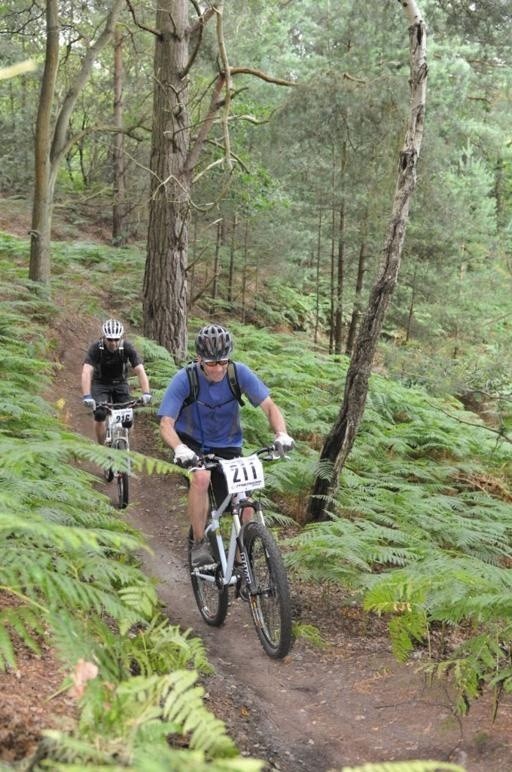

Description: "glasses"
206 360 227 367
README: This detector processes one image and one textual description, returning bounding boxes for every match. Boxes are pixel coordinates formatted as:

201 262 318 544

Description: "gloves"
275 433 294 456
84 394 96 411
141 393 152 405
173 444 197 473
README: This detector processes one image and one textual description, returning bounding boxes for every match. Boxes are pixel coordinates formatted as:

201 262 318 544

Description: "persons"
81 318 152 445
157 325 296 602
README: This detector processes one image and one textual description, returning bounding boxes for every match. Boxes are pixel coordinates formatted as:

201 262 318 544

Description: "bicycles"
84 387 151 509
177 442 294 660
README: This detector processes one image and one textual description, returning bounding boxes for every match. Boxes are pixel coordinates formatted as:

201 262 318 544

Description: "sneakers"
238 566 251 601
191 540 214 567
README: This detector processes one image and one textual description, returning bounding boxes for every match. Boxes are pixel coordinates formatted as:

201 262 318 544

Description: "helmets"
195 325 233 363
103 319 124 339
